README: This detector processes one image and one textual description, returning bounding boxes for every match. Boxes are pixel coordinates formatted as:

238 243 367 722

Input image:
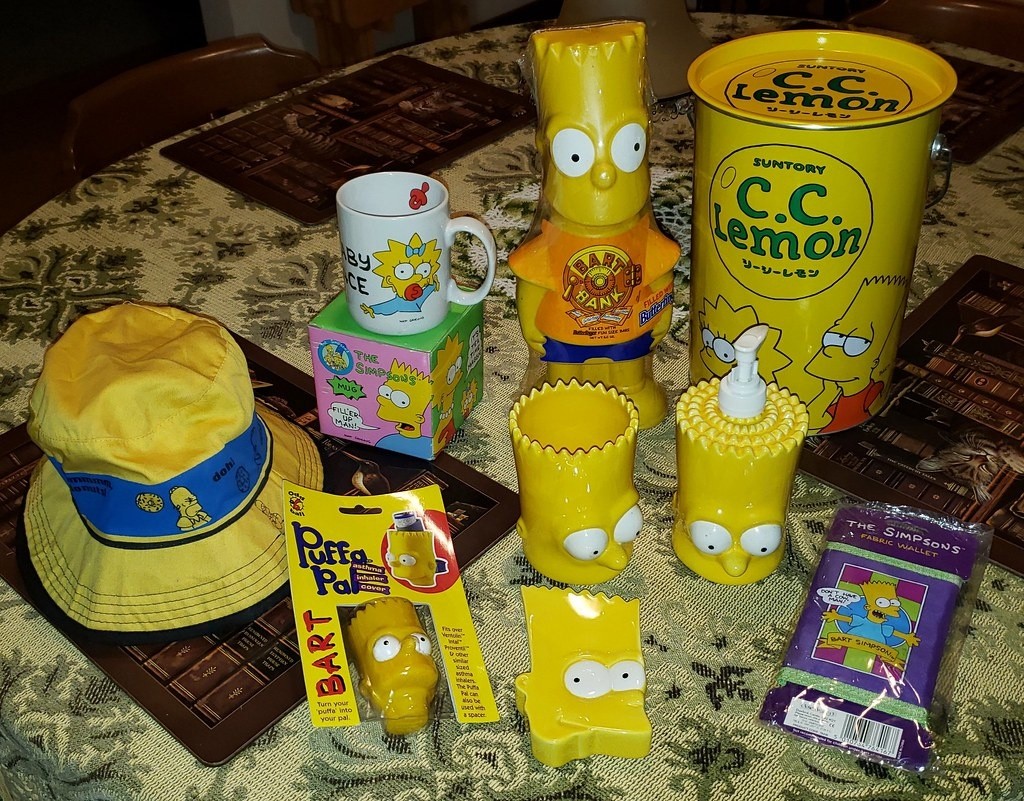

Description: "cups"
509 374 644 586
338 169 497 335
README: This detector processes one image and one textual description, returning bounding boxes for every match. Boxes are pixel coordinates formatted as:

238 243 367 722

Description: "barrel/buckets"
681 28 960 438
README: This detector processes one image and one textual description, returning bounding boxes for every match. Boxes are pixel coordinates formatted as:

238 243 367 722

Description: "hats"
552 1 713 108
12 305 335 647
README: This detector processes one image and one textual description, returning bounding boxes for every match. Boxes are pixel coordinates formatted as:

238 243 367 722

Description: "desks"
1 12 1023 801
